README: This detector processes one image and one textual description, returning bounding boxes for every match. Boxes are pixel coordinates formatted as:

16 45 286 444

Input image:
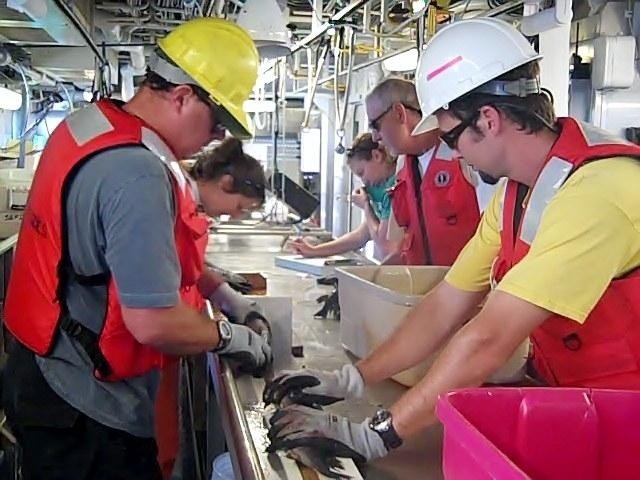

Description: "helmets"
157 17 259 140
410 17 543 136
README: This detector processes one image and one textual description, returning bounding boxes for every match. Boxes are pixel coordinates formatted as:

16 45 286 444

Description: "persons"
0 15 272 476
261 14 639 462
365 78 497 266
153 141 265 480
287 130 403 262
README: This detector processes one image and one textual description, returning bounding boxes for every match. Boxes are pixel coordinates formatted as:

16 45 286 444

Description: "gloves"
219 324 271 369
262 363 388 464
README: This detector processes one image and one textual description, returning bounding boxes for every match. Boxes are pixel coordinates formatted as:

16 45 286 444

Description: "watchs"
365 406 409 457
209 319 231 354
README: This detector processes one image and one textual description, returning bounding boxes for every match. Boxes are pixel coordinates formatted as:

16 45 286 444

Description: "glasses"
438 114 475 149
197 94 227 132
369 107 390 129
